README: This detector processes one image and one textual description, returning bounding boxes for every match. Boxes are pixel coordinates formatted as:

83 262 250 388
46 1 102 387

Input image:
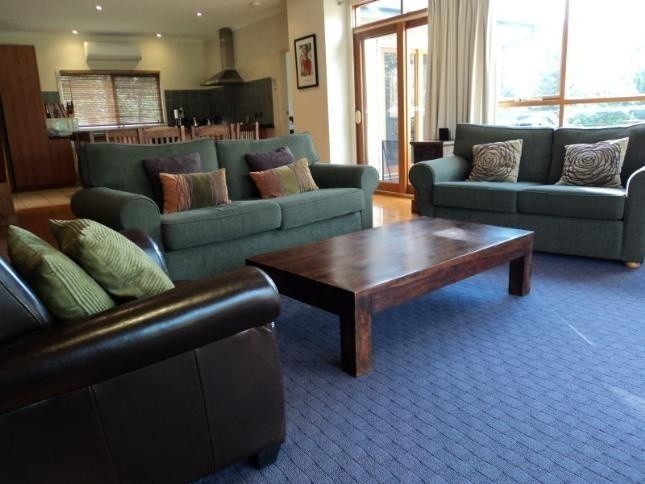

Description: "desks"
410 140 455 212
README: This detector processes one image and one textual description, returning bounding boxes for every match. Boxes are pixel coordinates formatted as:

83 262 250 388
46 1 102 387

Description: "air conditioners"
83 40 144 60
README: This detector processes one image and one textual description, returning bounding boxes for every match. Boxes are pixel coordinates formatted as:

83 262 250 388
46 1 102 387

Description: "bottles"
192 115 213 127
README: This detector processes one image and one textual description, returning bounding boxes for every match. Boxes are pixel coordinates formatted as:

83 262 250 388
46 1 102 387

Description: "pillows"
466 135 524 185
47 215 179 299
555 135 630 187
4 225 115 326
156 166 231 213
242 143 297 197
141 151 204 206
245 157 320 201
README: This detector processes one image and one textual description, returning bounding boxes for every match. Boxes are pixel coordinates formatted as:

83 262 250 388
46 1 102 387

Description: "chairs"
73 119 262 143
1 227 284 484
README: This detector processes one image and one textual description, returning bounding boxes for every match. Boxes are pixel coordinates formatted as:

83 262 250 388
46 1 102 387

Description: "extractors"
199 27 245 86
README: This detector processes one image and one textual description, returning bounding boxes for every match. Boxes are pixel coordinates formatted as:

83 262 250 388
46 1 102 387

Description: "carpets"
201 255 645 481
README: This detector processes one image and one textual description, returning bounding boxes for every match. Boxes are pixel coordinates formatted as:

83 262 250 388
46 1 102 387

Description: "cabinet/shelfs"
0 42 77 193
165 77 284 134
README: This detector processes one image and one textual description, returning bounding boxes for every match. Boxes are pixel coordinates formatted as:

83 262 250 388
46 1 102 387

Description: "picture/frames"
295 31 317 91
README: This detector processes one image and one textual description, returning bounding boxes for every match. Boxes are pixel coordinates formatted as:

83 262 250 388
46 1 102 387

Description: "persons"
299 44 312 75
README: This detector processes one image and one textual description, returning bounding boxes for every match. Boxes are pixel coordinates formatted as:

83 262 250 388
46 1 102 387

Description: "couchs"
66 132 381 278
408 121 645 263
